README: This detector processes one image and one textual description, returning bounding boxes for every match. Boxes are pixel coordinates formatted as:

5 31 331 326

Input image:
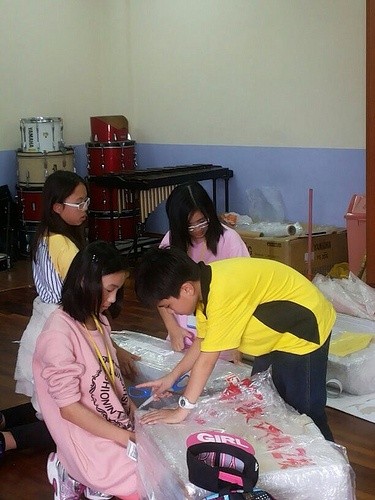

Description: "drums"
88 115 146 243
16 118 74 250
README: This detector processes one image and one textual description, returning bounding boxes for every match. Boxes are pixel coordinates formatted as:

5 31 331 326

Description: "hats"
185 431 259 495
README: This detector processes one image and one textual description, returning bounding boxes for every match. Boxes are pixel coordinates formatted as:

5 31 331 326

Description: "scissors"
128 374 189 409
185 331 195 348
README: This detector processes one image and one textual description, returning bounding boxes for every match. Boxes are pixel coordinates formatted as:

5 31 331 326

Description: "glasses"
64 198 90 211
187 219 209 232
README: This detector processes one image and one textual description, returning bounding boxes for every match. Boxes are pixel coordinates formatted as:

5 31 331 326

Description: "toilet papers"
271 224 296 237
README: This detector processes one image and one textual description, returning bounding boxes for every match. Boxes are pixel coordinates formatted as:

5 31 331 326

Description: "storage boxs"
239 223 348 282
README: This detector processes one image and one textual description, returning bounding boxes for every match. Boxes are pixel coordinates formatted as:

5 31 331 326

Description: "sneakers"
83 485 113 500
47 452 87 500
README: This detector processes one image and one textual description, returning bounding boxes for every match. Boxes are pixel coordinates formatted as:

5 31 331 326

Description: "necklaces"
82 313 116 392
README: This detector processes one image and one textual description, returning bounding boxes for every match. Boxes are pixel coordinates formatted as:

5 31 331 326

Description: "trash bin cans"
344 194 368 283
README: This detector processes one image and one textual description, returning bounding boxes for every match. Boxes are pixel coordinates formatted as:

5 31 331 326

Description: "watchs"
178 395 198 409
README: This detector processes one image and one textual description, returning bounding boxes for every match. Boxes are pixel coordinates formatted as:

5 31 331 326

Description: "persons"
134 248 337 443
157 182 250 364
14 170 139 420
32 241 191 500
